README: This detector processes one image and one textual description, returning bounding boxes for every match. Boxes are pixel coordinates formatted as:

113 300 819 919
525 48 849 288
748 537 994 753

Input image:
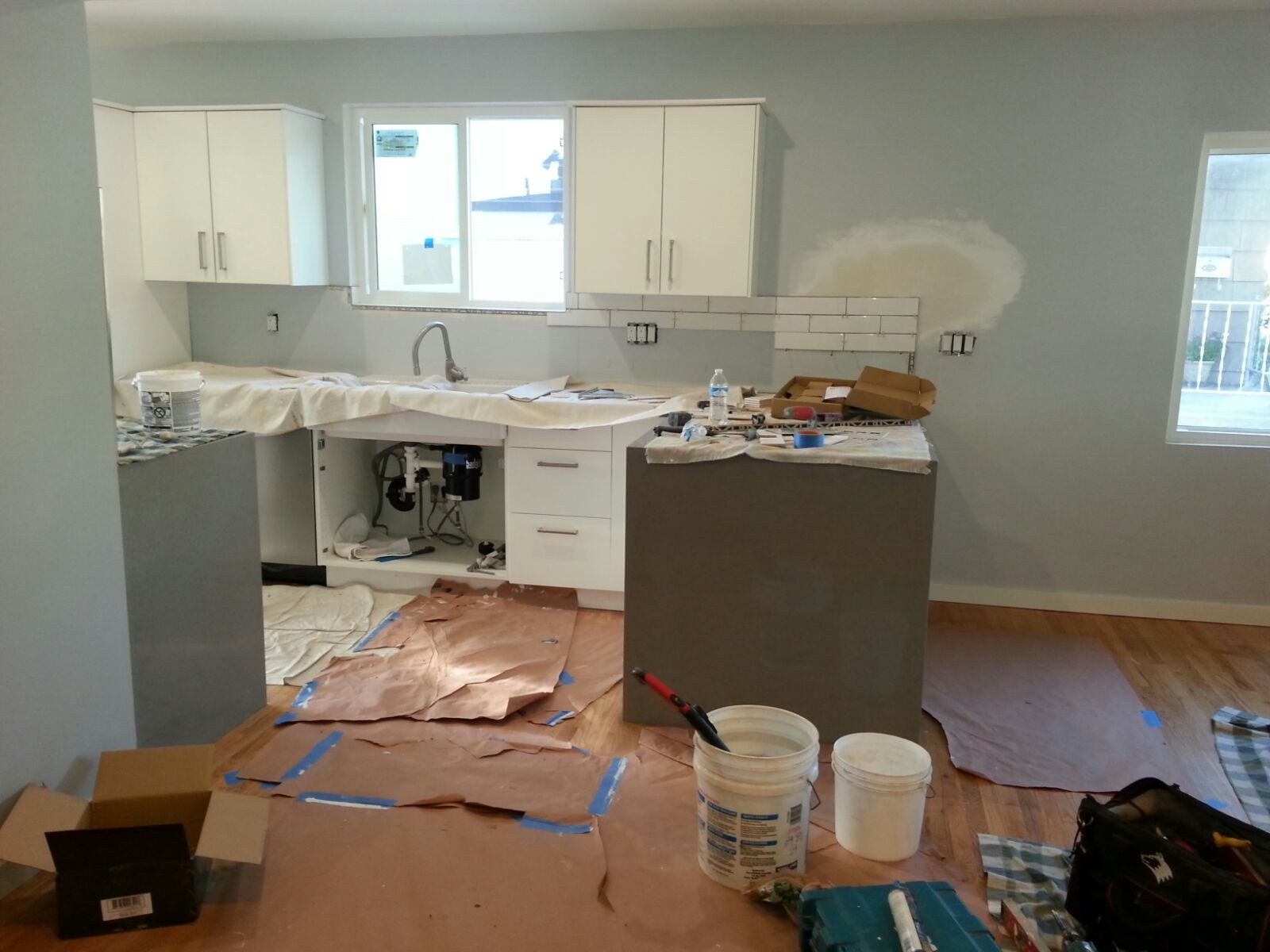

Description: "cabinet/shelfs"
503 424 626 594
565 96 768 304
134 103 327 288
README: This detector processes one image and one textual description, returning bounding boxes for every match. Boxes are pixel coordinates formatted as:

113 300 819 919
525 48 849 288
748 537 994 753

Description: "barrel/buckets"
831 732 937 863
692 704 822 893
132 369 206 440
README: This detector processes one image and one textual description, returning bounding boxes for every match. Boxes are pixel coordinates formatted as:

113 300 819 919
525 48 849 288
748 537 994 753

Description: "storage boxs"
769 365 939 422
0 738 276 942
792 879 1004 952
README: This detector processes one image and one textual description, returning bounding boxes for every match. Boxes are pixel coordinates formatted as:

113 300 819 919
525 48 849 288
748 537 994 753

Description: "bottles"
680 419 708 442
709 368 729 426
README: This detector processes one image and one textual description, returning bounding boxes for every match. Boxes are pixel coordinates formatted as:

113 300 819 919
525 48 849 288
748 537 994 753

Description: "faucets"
412 322 469 383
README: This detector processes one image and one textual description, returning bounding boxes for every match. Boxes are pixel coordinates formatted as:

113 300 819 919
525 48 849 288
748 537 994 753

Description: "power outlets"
265 315 278 332
647 325 657 343
626 323 637 343
637 326 647 343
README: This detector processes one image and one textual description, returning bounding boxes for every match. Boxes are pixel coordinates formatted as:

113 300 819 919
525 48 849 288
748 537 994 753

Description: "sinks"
419 381 524 396
334 374 429 390
